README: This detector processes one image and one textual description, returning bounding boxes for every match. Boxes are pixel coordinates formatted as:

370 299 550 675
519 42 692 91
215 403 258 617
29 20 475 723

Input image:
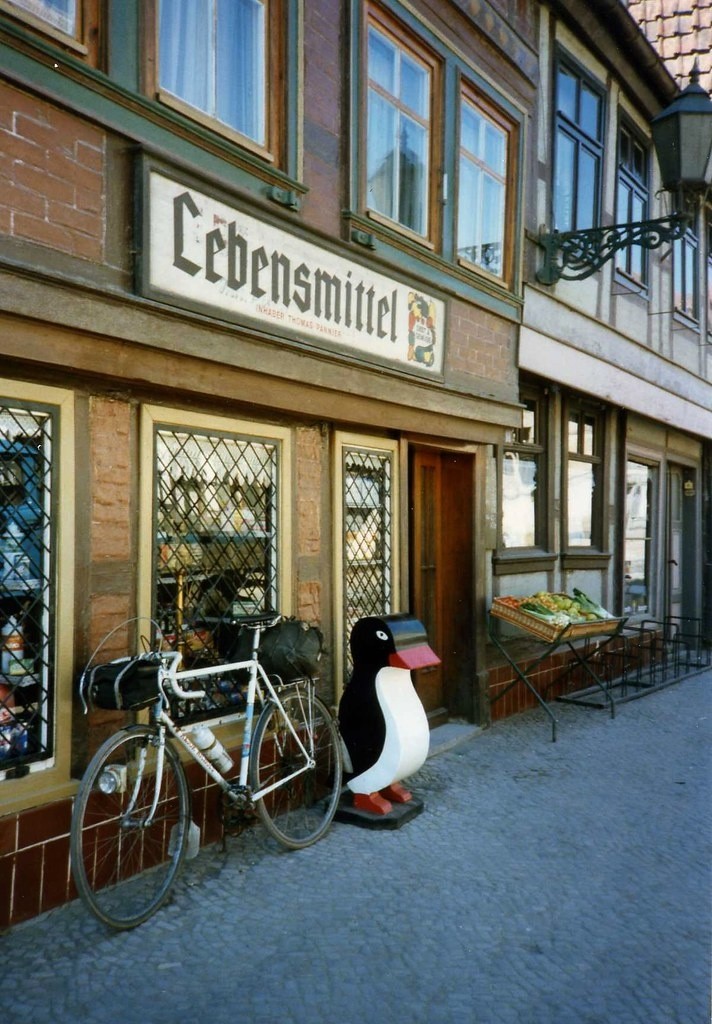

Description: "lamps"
532 55 712 286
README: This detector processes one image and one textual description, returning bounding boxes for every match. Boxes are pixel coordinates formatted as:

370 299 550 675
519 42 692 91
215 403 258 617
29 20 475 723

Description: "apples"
538 592 596 621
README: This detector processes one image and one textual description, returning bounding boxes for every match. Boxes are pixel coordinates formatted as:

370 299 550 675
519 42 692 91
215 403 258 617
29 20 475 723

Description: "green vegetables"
519 602 556 624
571 589 598 616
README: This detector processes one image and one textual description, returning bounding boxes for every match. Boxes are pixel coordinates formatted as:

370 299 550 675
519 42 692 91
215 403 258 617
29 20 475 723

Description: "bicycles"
67 607 344 932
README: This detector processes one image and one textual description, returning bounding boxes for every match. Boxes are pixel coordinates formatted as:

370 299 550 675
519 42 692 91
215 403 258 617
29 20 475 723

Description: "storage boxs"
491 595 620 643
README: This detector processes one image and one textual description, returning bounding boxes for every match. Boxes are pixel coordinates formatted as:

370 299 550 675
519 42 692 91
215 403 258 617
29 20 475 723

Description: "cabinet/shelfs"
0 577 45 687
154 530 268 623
